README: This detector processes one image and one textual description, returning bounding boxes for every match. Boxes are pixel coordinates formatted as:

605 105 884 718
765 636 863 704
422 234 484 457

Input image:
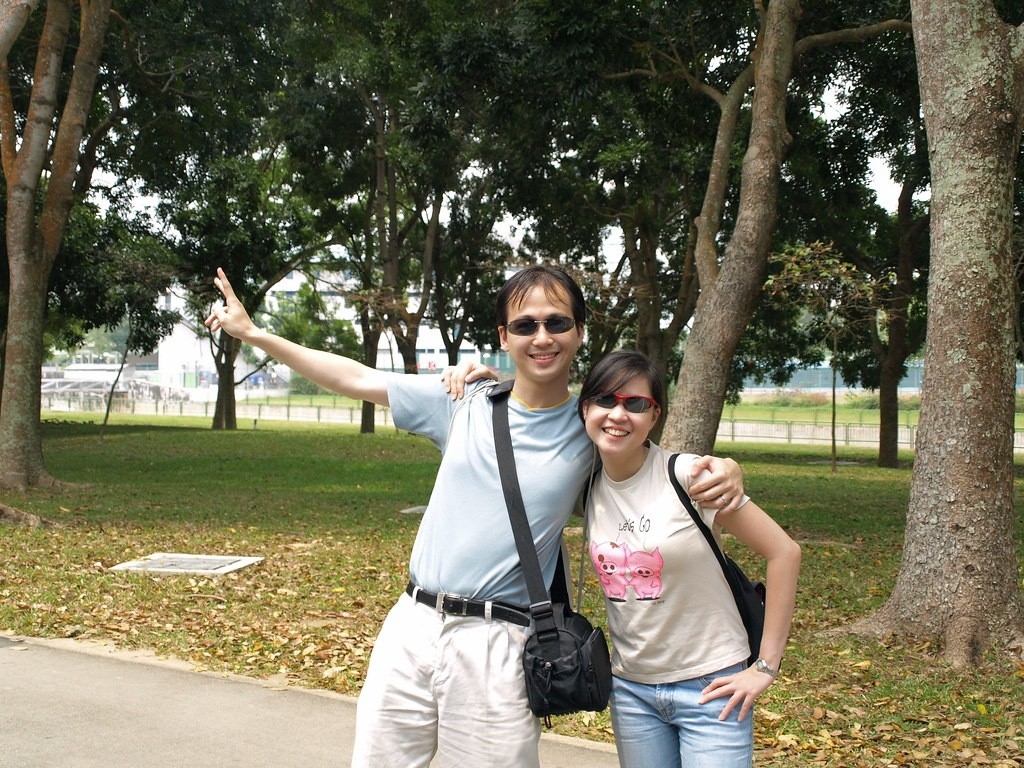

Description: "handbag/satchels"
722 551 782 673
521 600 611 719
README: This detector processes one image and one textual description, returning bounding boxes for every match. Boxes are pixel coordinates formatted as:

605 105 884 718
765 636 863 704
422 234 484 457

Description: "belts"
406 582 531 627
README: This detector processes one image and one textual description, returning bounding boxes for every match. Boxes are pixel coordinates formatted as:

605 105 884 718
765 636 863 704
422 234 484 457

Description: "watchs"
754 659 781 680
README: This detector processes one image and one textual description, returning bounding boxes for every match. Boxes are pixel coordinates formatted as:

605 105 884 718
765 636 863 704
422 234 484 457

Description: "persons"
204 266 802 768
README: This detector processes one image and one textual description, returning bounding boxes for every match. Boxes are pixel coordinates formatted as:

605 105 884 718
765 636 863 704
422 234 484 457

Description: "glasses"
505 316 581 335
594 389 661 413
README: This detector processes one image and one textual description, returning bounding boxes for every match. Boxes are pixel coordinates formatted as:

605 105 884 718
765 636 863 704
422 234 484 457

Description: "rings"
721 494 727 504
